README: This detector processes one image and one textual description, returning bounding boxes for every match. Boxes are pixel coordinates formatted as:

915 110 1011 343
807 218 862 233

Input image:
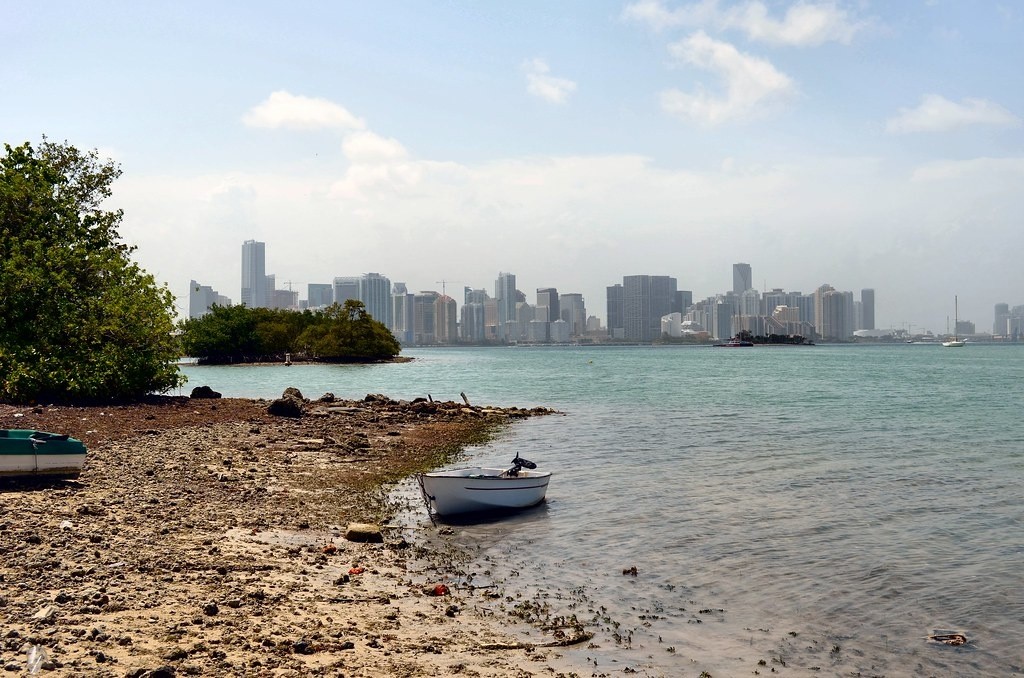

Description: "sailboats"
942 293 968 347
711 304 753 347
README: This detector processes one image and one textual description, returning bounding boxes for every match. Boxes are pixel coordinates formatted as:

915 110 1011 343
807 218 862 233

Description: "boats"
412 449 553 525
0 428 88 481
